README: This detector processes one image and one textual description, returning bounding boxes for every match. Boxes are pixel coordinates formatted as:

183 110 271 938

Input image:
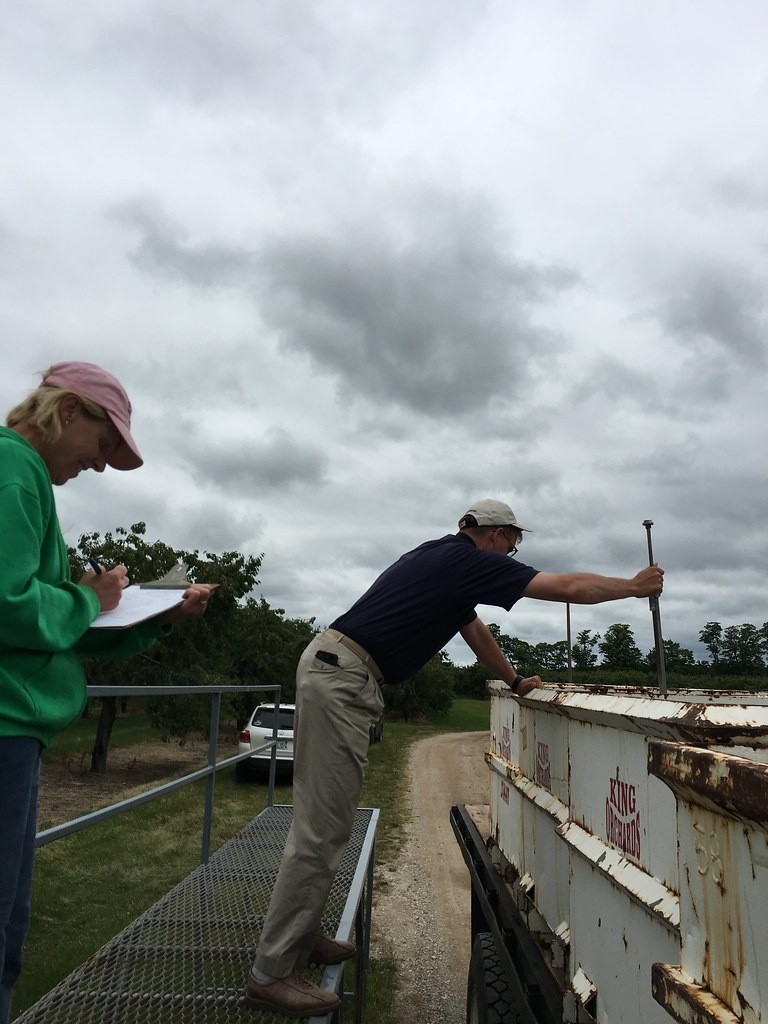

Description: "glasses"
492 529 518 558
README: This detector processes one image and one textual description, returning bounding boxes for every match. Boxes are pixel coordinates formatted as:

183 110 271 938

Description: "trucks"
449 679 768 1023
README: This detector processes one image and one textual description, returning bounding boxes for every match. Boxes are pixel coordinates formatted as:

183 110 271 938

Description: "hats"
39 362 143 471
458 498 533 533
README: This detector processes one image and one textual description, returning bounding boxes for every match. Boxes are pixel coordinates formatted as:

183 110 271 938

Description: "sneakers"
310 931 357 965
245 967 342 1017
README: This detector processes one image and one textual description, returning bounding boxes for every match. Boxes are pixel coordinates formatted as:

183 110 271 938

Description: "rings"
200 601 207 604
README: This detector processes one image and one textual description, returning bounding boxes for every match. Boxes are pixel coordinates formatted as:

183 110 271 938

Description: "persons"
1 359 214 1024
245 499 664 1014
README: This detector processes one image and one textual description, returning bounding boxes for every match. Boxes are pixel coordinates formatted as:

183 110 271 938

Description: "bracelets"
512 676 524 694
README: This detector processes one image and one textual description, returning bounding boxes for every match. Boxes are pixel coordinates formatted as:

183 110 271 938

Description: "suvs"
235 702 296 784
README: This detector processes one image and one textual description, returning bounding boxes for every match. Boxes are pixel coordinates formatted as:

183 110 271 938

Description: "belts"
327 629 388 692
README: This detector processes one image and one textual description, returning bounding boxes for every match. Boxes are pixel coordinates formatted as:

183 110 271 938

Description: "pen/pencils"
88 558 102 575
